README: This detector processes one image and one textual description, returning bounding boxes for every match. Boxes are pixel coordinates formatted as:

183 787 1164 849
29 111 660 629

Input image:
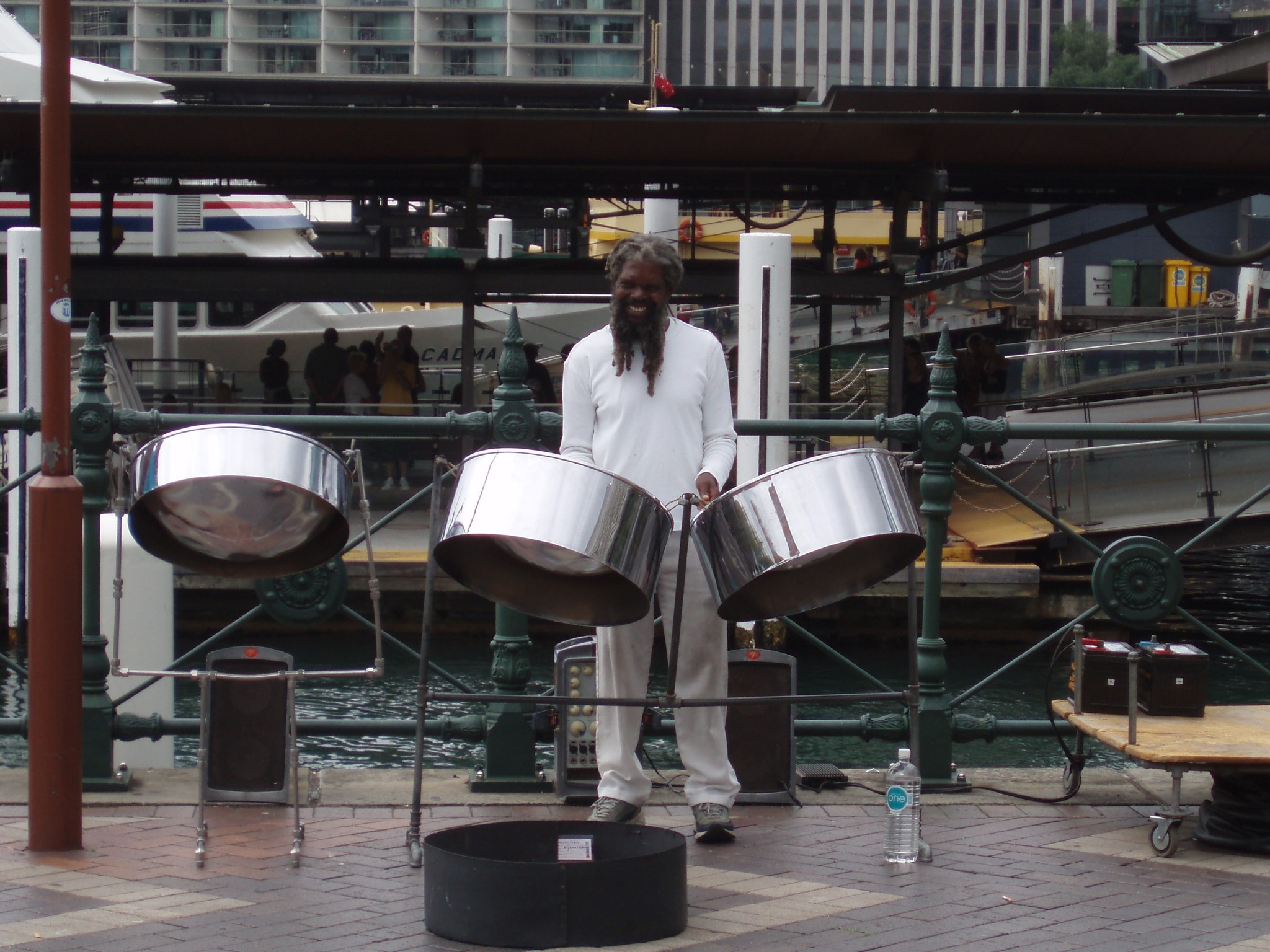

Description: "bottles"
882 748 922 864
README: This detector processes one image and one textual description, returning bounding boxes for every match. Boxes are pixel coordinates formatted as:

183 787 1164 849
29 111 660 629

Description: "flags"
653 64 675 100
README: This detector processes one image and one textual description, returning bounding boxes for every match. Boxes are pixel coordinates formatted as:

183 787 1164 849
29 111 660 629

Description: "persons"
723 345 739 418
904 333 1008 463
947 234 971 306
853 247 881 319
524 344 556 412
560 343 576 362
303 326 422 492
202 382 241 412
156 395 179 415
259 340 294 416
554 230 741 839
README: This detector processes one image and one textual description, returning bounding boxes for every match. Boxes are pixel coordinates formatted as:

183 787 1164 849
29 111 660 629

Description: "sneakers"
691 802 736 842
586 796 642 823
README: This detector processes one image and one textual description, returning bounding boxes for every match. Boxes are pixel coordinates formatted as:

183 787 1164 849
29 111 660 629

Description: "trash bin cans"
1106 259 1136 308
1163 259 1192 308
1189 265 1210 307
1137 259 1163 306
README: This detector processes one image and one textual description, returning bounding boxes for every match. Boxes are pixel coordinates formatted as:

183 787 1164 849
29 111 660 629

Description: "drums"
127 422 354 580
689 448 926 622
433 448 674 627
